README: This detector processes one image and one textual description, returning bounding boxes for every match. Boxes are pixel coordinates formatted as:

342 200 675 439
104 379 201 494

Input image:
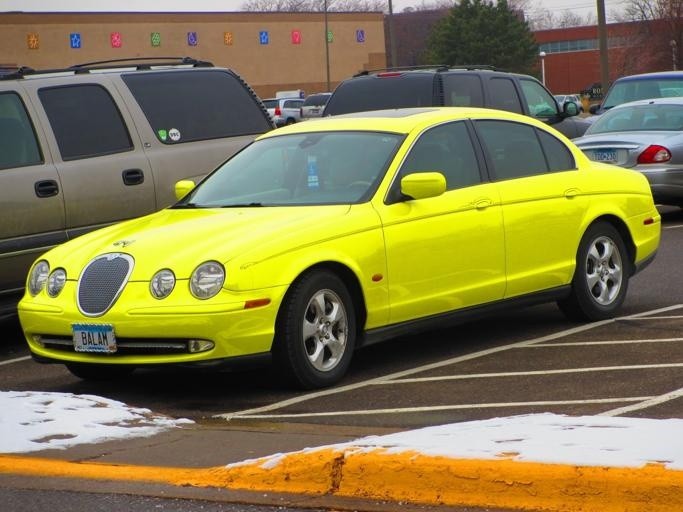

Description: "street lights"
670 40 677 70
540 52 546 86
